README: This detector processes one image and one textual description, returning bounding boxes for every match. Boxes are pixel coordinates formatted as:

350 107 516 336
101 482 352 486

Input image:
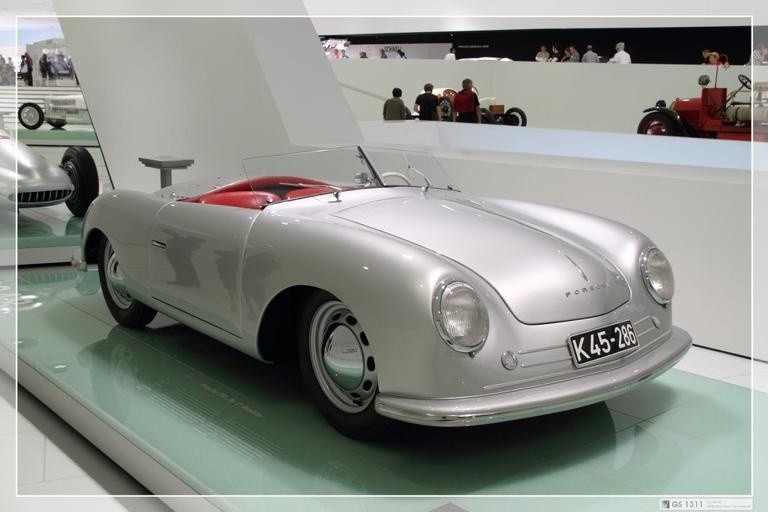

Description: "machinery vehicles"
637 74 767 143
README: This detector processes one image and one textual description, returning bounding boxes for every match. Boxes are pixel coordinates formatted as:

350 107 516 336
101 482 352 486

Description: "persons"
398 52 406 59
359 52 369 59
581 45 598 64
443 47 456 62
331 48 339 59
341 50 349 59
0 47 78 88
380 48 388 59
744 46 768 67
608 42 632 66
535 44 550 64
700 49 711 66
566 45 580 63
396 49 407 60
559 48 571 62
547 44 561 63
700 49 723 66
382 87 406 121
451 78 481 123
412 83 444 121
